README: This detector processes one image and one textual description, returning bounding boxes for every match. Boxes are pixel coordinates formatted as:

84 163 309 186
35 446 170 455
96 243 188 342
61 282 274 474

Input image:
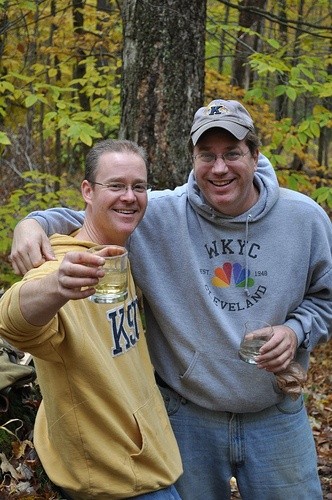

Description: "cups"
239 320 274 364
87 245 130 304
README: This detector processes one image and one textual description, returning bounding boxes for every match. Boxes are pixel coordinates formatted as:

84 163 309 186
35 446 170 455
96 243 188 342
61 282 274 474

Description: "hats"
190 100 254 146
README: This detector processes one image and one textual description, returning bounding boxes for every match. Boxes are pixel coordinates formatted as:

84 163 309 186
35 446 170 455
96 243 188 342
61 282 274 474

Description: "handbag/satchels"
0 338 37 415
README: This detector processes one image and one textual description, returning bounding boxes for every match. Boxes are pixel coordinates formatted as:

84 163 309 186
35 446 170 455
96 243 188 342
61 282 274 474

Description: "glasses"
87 180 153 193
192 150 250 161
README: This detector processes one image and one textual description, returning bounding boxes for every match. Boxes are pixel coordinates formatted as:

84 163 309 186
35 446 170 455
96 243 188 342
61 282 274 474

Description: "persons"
8 98 332 500
0 140 184 500
